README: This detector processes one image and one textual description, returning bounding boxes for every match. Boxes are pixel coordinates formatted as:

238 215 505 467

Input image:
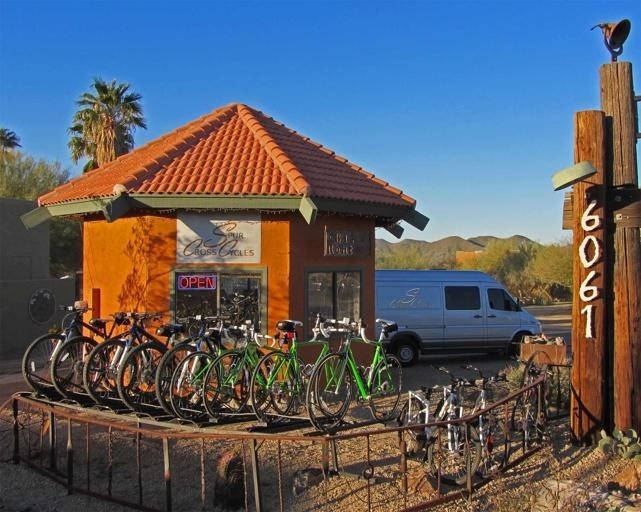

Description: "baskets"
457 384 480 409
487 385 510 403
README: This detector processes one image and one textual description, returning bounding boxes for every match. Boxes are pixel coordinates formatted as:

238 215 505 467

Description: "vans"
374 269 548 370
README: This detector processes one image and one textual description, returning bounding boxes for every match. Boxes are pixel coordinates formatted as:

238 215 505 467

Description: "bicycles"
174 289 260 340
20 300 351 434
305 312 402 432
398 359 554 484
